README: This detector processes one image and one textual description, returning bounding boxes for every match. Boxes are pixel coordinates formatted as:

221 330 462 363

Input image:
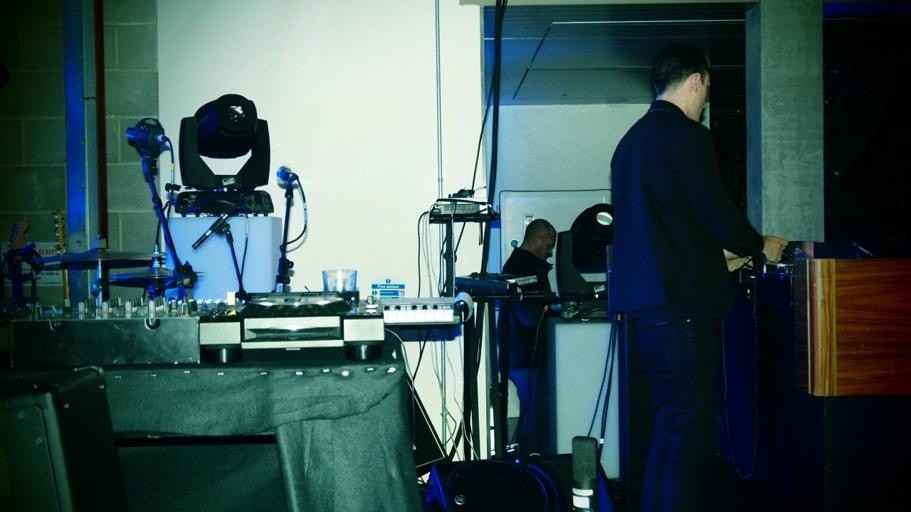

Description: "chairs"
161 96 279 307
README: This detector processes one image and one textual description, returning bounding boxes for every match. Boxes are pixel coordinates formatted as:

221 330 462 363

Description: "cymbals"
34 249 164 262
100 268 194 282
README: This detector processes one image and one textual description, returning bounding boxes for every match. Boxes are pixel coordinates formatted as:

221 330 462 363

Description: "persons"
609 41 789 512
496 218 557 481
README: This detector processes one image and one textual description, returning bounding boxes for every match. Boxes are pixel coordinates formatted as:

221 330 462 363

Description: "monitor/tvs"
556 230 608 295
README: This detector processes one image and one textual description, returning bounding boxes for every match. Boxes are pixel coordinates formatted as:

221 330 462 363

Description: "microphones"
570 435 599 511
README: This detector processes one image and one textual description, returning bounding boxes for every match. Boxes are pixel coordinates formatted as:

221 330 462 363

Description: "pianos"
381 298 455 325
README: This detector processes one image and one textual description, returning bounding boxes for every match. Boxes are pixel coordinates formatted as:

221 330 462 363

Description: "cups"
322 269 356 306
